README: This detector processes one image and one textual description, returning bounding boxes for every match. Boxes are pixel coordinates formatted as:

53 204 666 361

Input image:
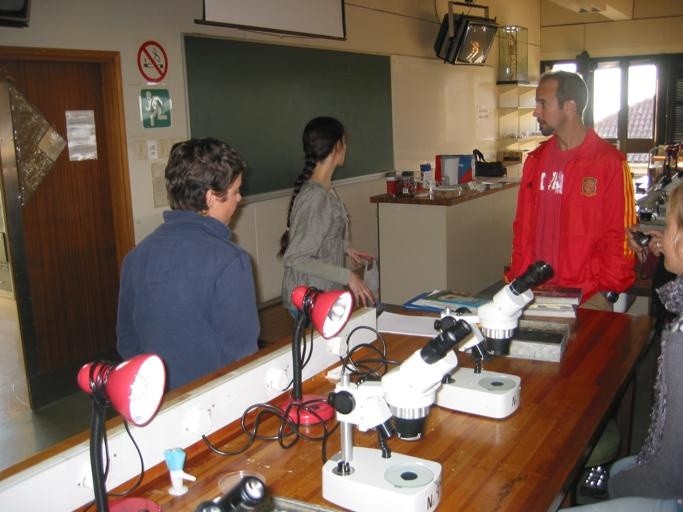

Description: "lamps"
75 351 171 511
281 282 355 426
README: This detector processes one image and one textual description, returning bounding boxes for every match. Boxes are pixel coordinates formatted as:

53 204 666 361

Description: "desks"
610 169 682 322
0 300 660 512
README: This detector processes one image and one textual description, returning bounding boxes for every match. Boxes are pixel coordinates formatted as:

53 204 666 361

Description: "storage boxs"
496 149 530 164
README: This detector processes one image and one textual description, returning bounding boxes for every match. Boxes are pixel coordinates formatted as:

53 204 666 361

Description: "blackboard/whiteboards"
181 32 394 206
387 52 498 176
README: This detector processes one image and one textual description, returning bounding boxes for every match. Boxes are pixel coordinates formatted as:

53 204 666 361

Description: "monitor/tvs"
432 12 500 67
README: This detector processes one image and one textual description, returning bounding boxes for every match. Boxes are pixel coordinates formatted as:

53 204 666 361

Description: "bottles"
420 163 432 189
402 171 411 186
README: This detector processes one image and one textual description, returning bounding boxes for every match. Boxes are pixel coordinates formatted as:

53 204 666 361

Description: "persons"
504 71 637 305
641 230 664 258
115 136 260 395
554 182 682 512
277 116 377 332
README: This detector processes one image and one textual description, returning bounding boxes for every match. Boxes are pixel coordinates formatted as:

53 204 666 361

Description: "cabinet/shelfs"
495 84 546 144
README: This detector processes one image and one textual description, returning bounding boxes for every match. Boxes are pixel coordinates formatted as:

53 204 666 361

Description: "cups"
639 207 653 222
386 176 399 195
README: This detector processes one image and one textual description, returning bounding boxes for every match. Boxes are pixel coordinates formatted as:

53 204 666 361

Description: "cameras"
632 231 652 247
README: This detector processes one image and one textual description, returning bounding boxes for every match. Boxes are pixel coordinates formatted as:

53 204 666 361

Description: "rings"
656 242 660 248
